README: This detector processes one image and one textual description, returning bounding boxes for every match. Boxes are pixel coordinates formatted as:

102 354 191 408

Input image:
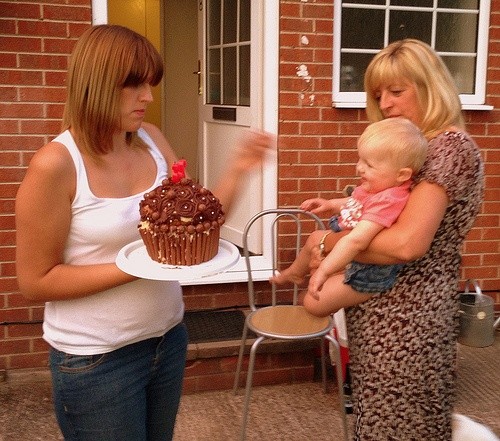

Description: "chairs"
231 208 348 441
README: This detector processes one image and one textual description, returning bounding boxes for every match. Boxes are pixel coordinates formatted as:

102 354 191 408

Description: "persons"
269 117 427 317
310 39 484 441
16 24 270 441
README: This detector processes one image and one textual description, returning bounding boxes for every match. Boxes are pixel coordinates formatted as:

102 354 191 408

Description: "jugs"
459 277 500 348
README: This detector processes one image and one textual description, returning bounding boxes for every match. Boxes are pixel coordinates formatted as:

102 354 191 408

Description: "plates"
115 235 241 281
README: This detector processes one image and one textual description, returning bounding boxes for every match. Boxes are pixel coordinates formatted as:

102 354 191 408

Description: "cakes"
137 160 225 266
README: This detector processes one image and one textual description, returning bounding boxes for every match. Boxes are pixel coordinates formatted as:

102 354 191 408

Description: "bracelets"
319 231 333 256
